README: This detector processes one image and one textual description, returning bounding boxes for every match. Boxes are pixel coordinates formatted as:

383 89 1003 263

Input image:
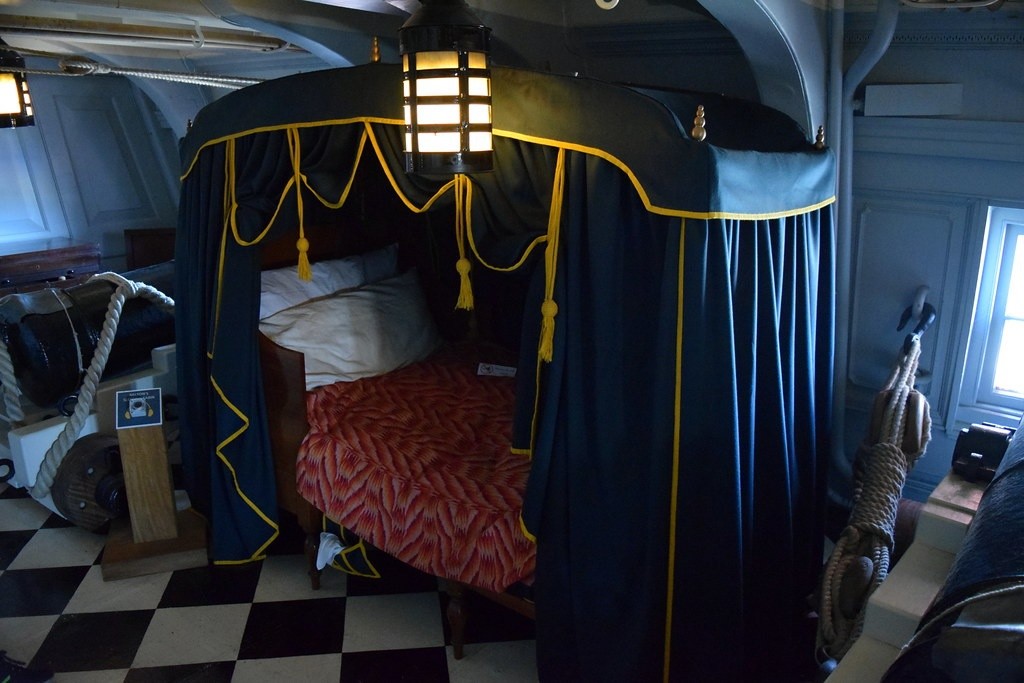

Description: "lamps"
397 0 495 175
0 37 35 129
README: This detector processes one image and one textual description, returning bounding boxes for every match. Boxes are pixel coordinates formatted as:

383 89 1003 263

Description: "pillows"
258 243 400 319
258 266 444 391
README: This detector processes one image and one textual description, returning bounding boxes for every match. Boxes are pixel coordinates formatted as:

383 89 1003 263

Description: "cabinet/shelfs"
0 236 101 299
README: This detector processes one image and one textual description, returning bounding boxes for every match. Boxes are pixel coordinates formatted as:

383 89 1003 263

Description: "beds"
259 328 544 660
